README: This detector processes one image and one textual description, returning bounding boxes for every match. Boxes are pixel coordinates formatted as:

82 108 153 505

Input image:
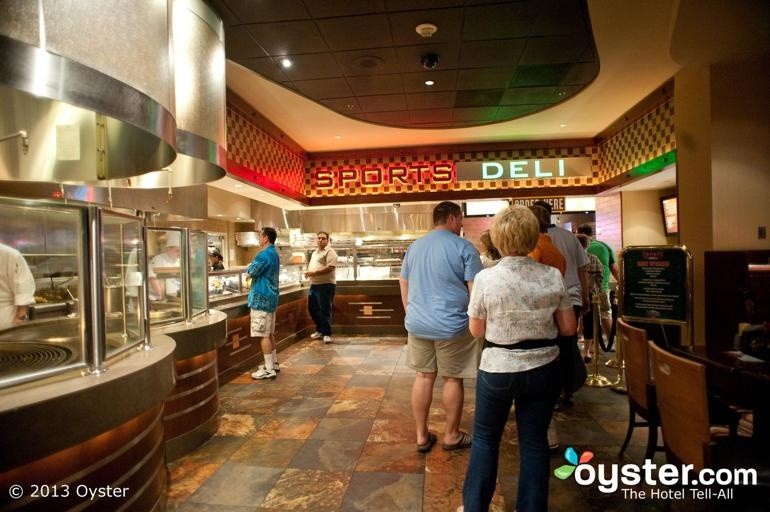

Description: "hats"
528 206 551 229
166 226 180 247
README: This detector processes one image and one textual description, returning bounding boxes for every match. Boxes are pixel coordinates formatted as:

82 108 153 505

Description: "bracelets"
16 311 30 321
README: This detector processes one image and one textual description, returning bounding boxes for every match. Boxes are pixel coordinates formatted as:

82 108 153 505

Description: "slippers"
442 432 473 451
417 432 437 453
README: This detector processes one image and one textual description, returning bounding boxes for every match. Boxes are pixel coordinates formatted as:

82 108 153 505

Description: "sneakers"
311 332 324 339
550 444 564 459
251 369 277 380
323 336 332 344
258 362 280 372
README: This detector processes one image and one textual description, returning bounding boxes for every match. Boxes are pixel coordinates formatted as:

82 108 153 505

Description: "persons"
455 202 579 512
245 225 282 381
1 240 39 332
148 231 183 301
303 229 338 343
399 199 484 453
478 201 622 412
207 251 224 271
123 245 160 312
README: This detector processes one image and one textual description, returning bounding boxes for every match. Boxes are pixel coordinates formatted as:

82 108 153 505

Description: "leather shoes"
584 352 594 364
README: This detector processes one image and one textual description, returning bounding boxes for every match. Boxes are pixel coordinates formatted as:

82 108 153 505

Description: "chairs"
615 310 769 511
